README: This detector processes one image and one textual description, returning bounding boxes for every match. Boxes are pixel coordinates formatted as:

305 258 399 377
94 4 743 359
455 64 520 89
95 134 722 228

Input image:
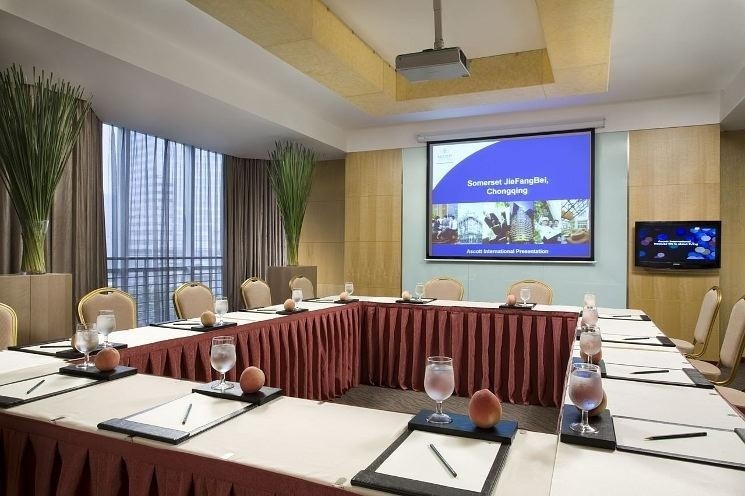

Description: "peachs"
580 349 602 363
402 292 412 300
588 389 607 416
340 293 348 301
201 311 216 326
284 299 295 311
240 367 265 393
95 348 119 371
507 295 517 305
469 388 502 429
71 335 75 348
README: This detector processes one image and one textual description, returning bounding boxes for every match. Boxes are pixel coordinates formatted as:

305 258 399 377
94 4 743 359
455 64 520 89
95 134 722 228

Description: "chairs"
682 293 745 387
715 381 745 411
668 284 726 359
422 276 466 302
287 271 315 301
1 300 21 353
171 280 217 321
237 275 275 308
76 286 136 336
503 277 554 306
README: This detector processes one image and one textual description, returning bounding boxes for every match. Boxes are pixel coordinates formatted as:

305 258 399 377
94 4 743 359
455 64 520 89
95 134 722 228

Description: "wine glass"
584 292 596 307
578 326 603 361
344 282 354 296
214 297 229 326
582 305 599 326
521 287 532 306
94 310 116 348
414 282 426 302
422 356 455 426
566 361 605 435
290 288 303 312
73 322 99 369
207 335 238 392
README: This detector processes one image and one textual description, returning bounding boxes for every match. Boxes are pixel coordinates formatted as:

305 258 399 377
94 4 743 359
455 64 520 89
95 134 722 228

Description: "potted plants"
0 61 94 273
258 136 317 270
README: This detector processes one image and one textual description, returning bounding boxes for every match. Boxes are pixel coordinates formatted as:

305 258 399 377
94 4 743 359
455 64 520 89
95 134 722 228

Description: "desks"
1 294 745 495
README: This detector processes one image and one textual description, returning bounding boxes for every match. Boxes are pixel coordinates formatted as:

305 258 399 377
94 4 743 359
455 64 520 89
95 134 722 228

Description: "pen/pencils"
621 337 650 340
40 345 72 348
173 323 200 325
430 443 457 480
256 309 276 312
180 403 193 424
316 299 333 301
612 315 631 318
25 379 45 394
643 431 707 440
629 370 669 374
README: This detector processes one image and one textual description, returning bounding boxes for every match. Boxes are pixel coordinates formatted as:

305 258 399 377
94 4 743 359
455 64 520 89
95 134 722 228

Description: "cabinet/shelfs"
1 271 72 344
266 263 318 306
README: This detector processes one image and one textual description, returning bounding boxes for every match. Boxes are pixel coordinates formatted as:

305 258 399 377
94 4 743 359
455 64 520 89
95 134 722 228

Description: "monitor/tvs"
633 220 721 276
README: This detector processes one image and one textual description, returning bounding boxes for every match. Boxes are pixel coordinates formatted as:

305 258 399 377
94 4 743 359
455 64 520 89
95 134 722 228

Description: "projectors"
396 46 470 83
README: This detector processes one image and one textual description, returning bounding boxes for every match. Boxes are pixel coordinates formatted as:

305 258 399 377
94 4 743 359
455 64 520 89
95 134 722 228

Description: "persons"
434 214 458 240
480 208 508 242
535 217 561 243
